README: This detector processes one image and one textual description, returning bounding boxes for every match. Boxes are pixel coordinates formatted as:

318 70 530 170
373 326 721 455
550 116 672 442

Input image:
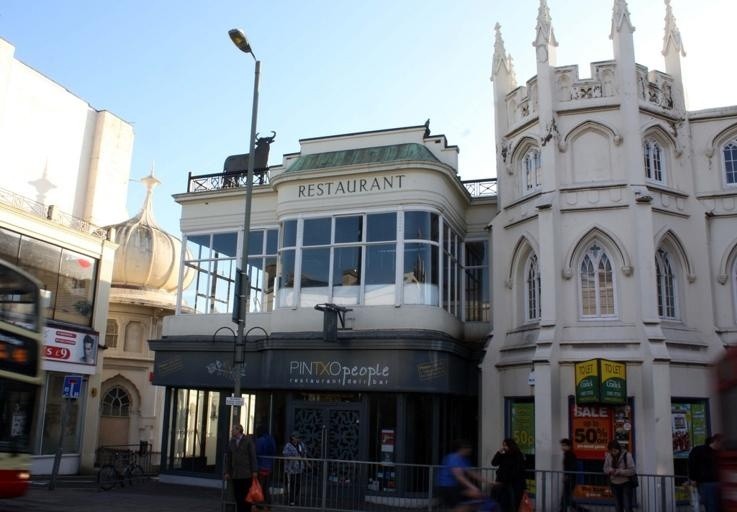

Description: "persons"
79 336 96 364
224 424 258 511
672 430 691 452
559 438 590 512
604 439 637 512
686 433 726 510
254 423 278 511
434 434 527 512
282 430 313 505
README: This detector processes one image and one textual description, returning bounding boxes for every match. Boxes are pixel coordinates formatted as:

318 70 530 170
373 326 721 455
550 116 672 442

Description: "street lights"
228 28 260 439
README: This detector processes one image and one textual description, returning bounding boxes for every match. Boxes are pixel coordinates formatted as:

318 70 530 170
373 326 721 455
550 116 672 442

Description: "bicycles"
97 448 144 491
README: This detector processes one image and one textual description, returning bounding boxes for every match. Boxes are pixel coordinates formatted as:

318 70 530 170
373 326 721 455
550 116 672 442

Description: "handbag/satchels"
628 470 639 488
303 455 313 473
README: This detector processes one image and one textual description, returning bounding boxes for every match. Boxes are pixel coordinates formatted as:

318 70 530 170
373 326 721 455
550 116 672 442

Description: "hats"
292 431 301 441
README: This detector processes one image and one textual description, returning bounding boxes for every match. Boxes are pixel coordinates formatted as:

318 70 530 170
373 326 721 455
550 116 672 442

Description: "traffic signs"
60 375 83 400
